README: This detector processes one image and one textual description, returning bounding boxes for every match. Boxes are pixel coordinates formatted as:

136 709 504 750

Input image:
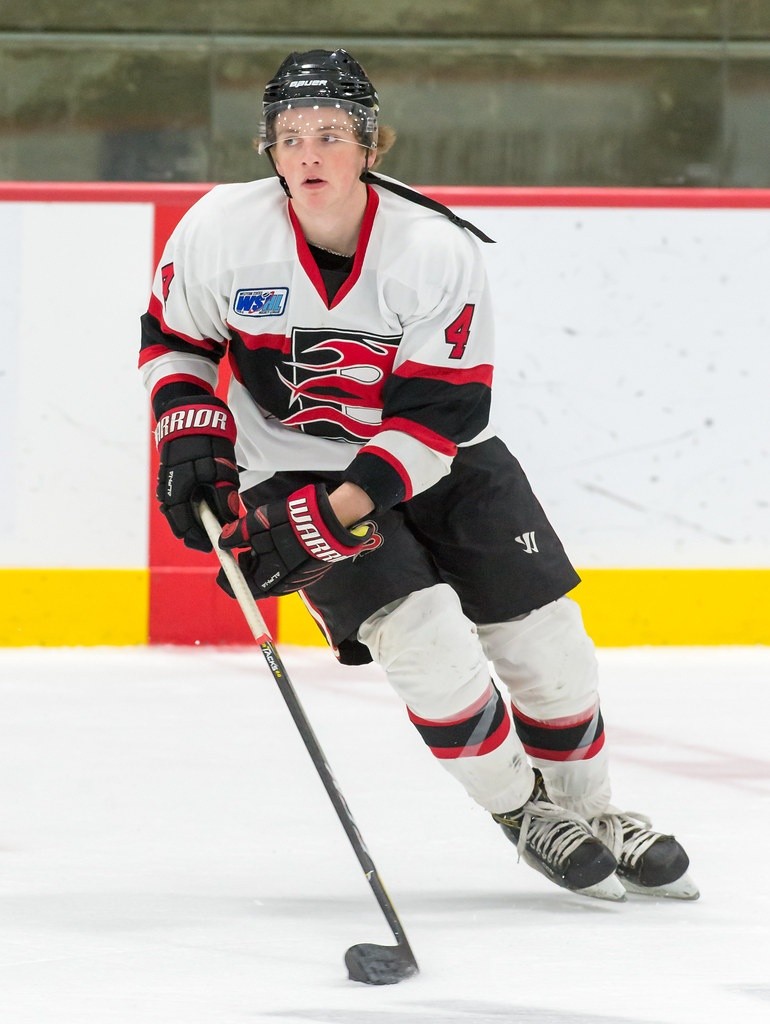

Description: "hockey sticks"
189 487 424 988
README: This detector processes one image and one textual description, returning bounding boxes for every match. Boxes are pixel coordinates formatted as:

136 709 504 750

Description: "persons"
138 49 699 903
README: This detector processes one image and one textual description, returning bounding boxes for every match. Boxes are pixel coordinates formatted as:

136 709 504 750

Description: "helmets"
262 48 381 155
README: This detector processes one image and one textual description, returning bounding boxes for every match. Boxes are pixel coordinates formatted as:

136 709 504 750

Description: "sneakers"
492 767 626 901
579 803 701 900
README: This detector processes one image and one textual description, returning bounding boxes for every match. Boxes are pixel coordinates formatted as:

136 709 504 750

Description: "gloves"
216 481 374 602
155 395 242 554
